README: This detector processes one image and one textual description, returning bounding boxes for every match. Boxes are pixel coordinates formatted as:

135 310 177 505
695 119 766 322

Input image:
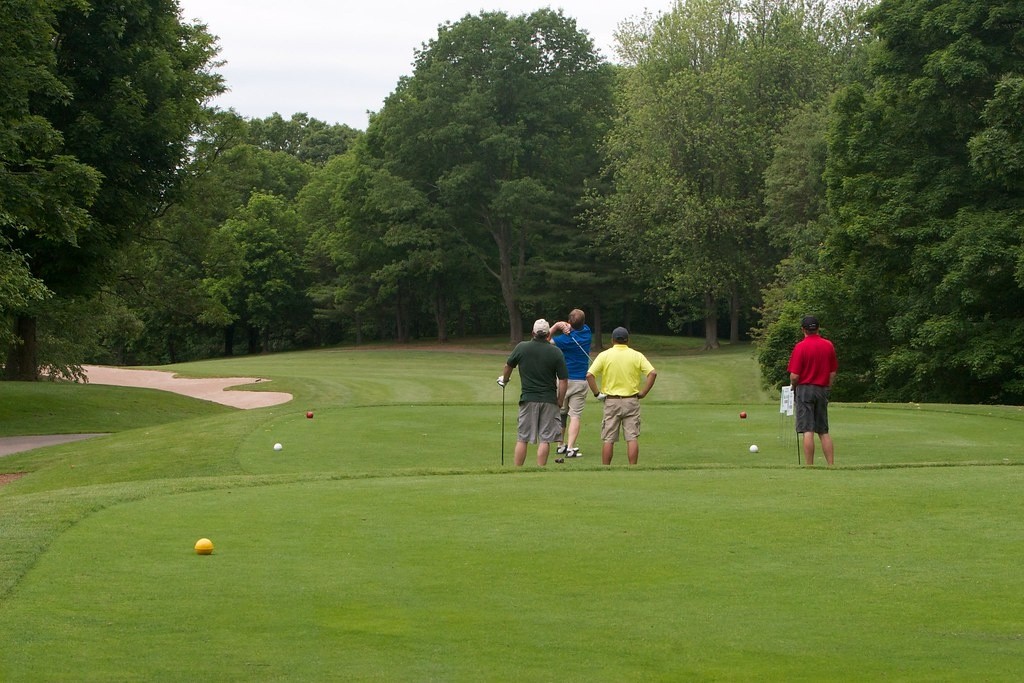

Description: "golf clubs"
792 384 801 466
497 379 508 467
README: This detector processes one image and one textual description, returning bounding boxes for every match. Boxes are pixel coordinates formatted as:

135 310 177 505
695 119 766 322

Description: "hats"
532 318 549 335
800 316 819 331
612 326 629 342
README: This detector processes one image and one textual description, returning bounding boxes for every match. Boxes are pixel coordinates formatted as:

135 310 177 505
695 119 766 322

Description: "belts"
606 394 638 399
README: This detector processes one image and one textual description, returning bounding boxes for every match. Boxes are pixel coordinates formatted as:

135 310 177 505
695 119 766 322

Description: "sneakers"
557 444 579 454
565 448 583 457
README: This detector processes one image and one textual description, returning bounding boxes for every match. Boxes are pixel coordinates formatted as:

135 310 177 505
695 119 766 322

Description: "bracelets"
594 392 600 397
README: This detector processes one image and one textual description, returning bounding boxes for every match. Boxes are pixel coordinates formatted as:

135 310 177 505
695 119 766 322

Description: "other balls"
739 411 747 419
306 412 313 418
749 444 759 453
273 443 282 451
194 537 213 555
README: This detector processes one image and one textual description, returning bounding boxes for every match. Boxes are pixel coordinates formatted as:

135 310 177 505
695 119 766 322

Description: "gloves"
496 376 511 383
594 392 607 403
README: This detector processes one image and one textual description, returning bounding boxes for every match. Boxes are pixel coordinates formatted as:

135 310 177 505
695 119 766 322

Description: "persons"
787 317 838 465
586 327 657 465
547 309 592 457
497 318 567 466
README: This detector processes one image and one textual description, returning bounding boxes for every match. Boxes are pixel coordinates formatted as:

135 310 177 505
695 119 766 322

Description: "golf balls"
567 330 594 364
554 458 564 464
